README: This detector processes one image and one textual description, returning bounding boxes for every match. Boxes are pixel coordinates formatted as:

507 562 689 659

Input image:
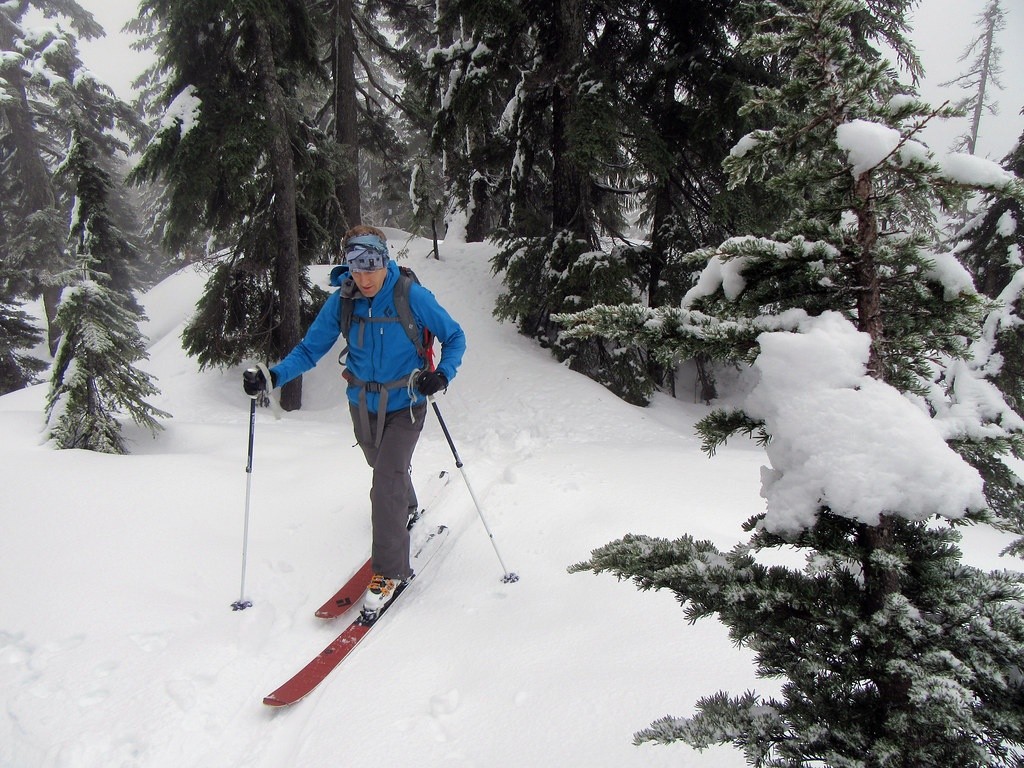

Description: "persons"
243 227 466 613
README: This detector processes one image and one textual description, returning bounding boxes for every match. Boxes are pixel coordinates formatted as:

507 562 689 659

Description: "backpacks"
340 266 435 390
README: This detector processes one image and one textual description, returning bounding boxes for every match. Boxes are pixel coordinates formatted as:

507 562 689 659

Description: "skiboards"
262 472 452 708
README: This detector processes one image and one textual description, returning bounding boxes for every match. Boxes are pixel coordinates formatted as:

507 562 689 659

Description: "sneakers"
363 573 401 611
406 513 414 528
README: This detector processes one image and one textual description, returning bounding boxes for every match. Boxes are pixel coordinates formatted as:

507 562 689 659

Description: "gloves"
416 370 449 395
243 368 277 399
